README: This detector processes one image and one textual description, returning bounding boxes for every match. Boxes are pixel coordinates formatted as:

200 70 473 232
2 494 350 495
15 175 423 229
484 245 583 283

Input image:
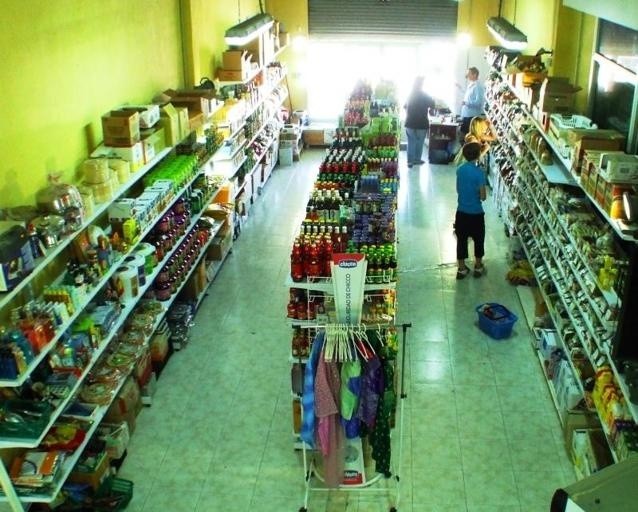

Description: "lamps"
622 192 638 222
486 0 527 50
223 0 274 45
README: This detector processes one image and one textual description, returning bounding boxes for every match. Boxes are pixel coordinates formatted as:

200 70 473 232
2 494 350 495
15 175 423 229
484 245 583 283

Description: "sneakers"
472 262 488 278
408 159 426 170
455 265 470 280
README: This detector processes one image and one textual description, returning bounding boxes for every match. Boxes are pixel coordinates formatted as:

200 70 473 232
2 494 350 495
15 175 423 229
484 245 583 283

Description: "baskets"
94 476 135 512
475 301 520 341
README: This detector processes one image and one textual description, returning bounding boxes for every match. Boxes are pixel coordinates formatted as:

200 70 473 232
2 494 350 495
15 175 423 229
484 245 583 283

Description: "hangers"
324 323 385 363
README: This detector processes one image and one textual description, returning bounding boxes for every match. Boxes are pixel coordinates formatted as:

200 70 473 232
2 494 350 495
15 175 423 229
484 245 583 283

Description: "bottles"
148 175 223 301
285 97 400 359
89 239 110 285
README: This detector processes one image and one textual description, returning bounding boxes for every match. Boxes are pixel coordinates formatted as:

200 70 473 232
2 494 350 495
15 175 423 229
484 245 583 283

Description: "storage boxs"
69 447 113 492
131 343 152 388
216 223 231 240
87 419 131 465
104 376 143 426
147 334 169 370
194 256 207 292
205 236 227 262
567 127 627 178
540 77 583 112
538 328 592 459
175 269 199 303
100 21 325 173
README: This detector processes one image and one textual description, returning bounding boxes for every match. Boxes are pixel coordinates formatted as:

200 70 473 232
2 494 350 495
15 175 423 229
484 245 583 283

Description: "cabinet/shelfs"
0 41 291 512
287 76 401 453
480 50 638 481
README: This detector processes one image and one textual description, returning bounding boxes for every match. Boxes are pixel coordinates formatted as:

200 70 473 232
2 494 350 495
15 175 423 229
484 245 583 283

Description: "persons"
455 66 484 149
453 143 488 280
402 74 443 169
457 116 499 169
